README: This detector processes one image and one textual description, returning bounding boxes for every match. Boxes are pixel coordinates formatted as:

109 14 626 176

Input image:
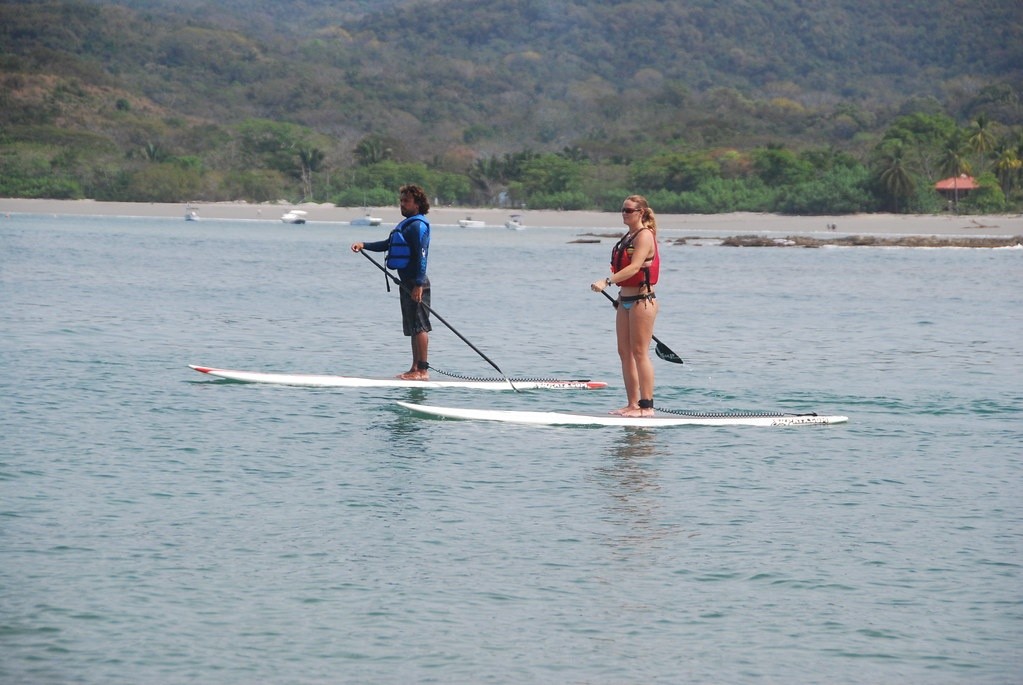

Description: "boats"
457 218 485 228
350 216 382 226
281 210 308 225
185 205 200 220
504 213 523 228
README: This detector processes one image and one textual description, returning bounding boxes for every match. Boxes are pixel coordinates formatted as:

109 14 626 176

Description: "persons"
591 195 660 416
352 185 432 380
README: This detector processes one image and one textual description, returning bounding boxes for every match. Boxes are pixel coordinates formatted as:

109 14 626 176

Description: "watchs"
607 278 611 286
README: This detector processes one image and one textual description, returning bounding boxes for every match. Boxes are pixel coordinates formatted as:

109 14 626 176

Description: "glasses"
621 207 642 214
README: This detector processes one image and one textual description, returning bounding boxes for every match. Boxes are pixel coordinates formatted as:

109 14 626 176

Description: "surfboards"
396 400 849 428
185 363 610 391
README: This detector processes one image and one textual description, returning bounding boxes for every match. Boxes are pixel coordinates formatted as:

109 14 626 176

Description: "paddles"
359 247 504 374
601 290 684 364
